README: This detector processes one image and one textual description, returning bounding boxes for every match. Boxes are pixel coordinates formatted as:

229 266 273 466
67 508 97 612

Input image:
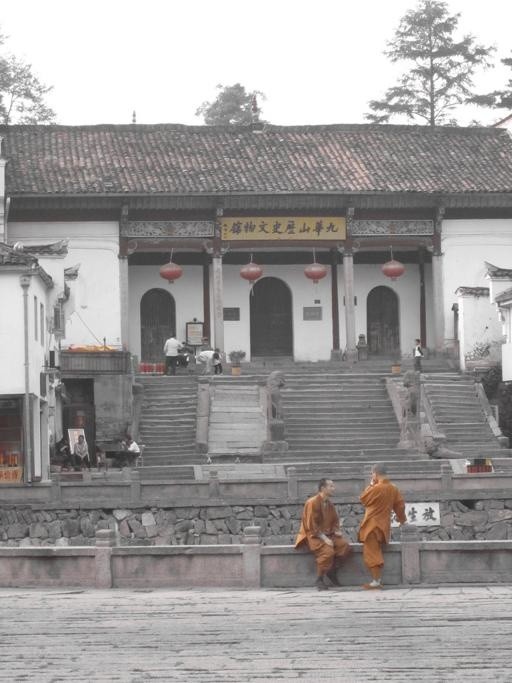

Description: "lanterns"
239 261 262 283
158 261 184 284
304 261 326 284
381 259 405 281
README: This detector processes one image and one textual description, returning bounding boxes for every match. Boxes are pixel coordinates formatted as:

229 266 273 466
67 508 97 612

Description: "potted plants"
390 348 402 374
228 349 246 376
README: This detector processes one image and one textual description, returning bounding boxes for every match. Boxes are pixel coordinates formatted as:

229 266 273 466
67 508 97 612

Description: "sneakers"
360 579 384 590
315 576 329 592
327 571 340 587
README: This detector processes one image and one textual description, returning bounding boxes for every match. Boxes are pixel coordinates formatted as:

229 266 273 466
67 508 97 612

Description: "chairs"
80 449 144 472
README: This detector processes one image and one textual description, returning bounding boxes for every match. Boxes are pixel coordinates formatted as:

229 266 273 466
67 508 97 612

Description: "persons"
292 476 352 591
55 436 75 471
162 332 183 375
214 347 223 374
75 434 92 473
412 338 423 372
355 460 409 590
197 350 221 374
118 433 141 468
